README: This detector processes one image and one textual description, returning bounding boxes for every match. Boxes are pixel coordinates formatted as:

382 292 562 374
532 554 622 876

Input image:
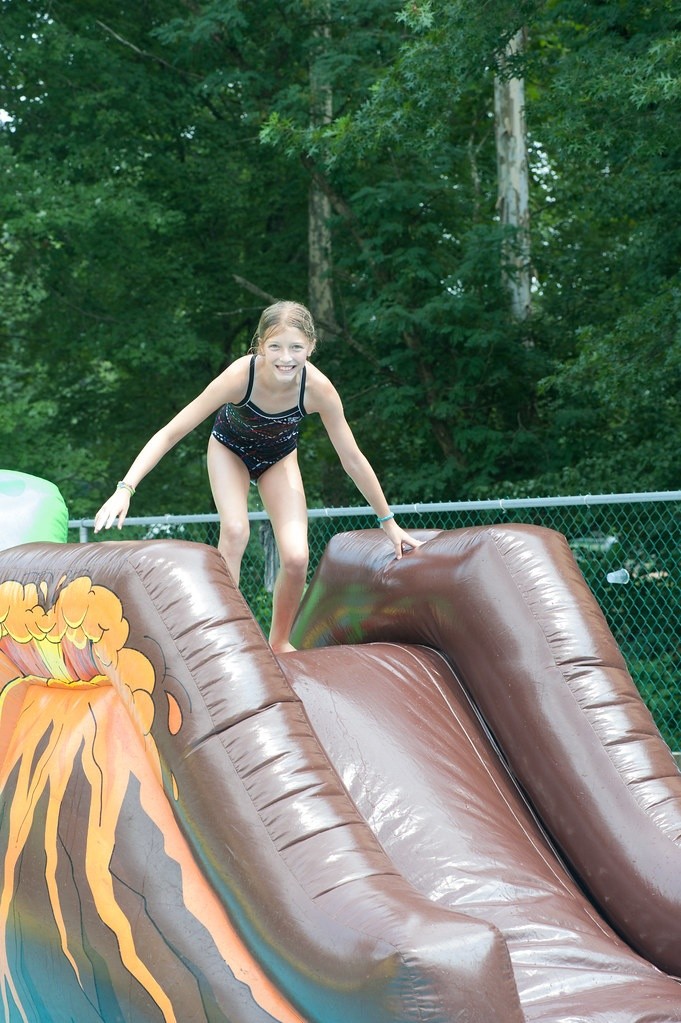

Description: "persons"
92 301 426 656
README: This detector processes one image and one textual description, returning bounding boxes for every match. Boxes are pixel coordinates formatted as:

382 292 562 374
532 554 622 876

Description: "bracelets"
117 481 136 497
377 512 394 522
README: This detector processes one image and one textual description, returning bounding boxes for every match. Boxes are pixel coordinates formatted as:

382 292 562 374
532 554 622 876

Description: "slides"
261 638 681 1023
